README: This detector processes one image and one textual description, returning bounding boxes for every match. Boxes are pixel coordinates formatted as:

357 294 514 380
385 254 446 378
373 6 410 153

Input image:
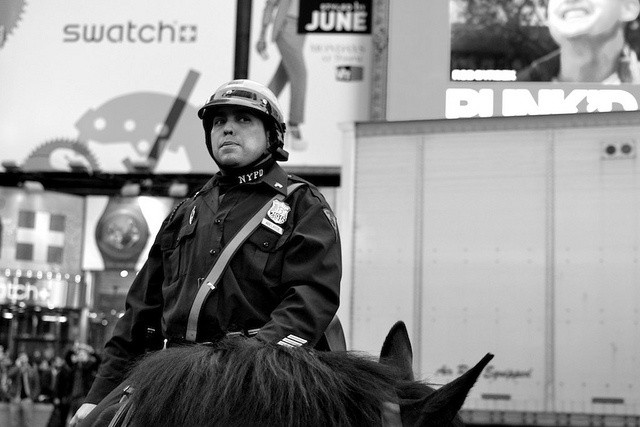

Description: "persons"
68 80 342 426
0 345 98 426
516 0 639 85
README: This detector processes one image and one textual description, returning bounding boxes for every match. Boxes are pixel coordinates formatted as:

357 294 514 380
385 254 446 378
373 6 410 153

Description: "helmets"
198 79 286 148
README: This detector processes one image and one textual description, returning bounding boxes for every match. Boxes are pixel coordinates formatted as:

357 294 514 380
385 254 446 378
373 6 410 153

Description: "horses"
76 320 494 427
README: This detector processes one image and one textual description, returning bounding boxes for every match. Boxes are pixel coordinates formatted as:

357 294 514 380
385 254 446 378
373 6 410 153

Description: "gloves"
69 404 97 427
